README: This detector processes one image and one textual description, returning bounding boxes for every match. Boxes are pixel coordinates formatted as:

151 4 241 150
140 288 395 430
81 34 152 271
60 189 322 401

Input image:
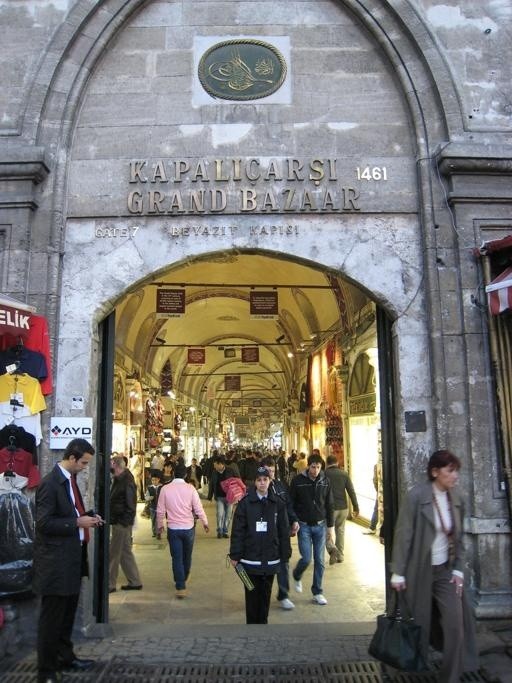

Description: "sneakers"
314 594 325 605
217 533 229 539
173 585 191 598
327 546 344 564
278 595 293 609
294 578 302 592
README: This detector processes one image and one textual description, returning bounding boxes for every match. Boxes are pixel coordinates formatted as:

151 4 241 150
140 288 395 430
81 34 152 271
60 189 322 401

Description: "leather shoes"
59 656 96 671
121 584 144 590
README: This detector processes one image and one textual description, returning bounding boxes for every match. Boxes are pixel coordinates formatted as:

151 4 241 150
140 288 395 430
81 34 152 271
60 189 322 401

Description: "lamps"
285 328 331 361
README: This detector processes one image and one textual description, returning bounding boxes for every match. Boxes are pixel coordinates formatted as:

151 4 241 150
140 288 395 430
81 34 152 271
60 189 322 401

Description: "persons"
32 439 108 683
390 450 480 683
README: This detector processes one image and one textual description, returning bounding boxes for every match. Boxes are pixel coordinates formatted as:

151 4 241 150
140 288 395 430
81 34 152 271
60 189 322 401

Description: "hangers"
2 330 26 496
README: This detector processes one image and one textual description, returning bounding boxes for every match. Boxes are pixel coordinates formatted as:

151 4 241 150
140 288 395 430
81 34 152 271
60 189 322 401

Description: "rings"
459 584 464 587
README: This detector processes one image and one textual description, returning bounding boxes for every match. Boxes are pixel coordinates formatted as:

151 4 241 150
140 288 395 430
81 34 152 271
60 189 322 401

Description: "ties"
70 474 91 542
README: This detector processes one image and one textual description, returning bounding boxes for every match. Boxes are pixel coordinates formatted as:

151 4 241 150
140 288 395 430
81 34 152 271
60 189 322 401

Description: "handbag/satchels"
366 588 424 669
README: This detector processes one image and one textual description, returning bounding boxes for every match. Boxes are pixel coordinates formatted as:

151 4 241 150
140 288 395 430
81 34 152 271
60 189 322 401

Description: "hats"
255 466 272 478
